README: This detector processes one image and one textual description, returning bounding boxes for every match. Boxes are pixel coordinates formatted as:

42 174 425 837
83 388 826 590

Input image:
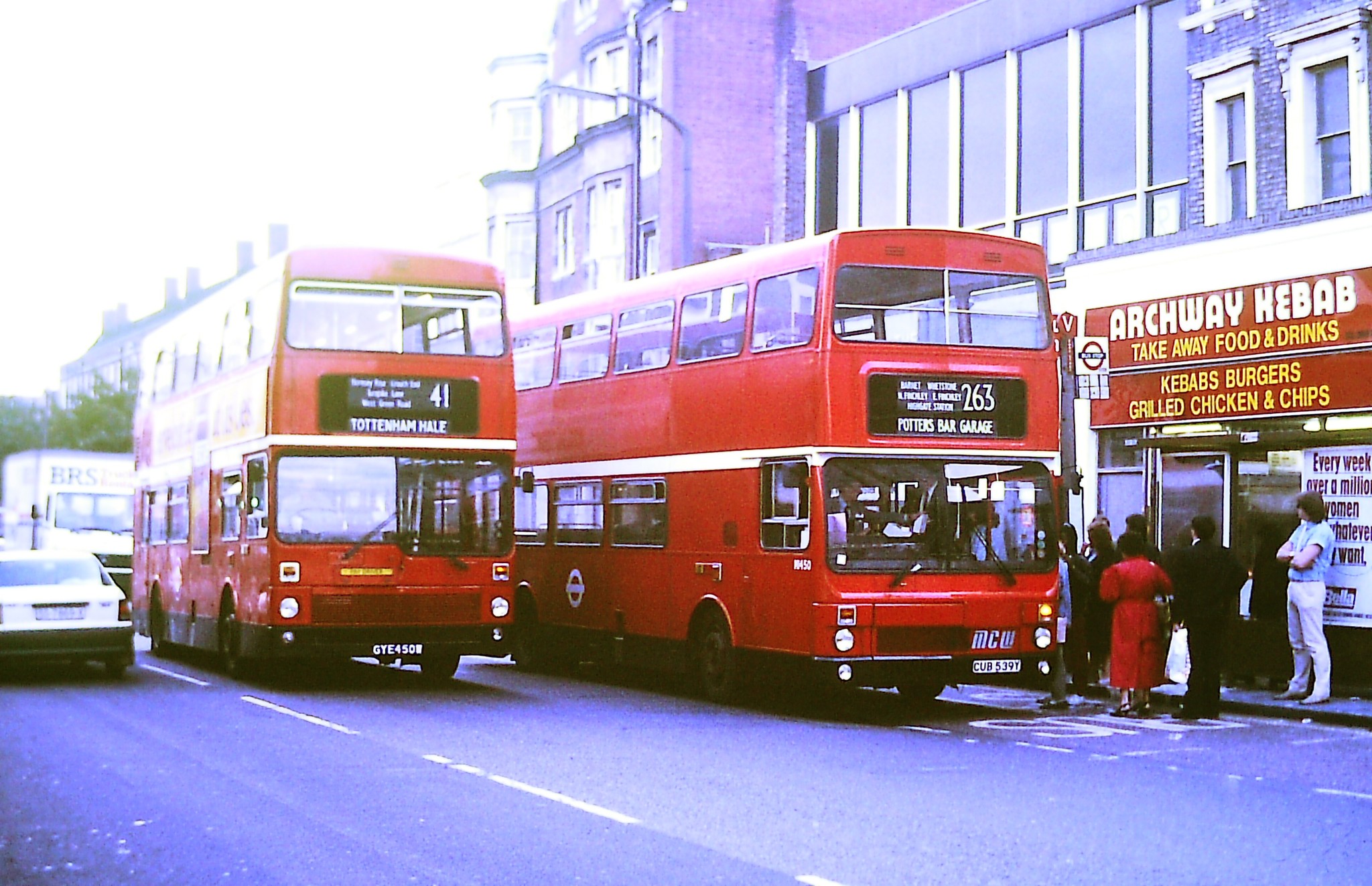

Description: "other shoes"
1300 694 1332 706
1273 689 1308 701
1109 701 1151 719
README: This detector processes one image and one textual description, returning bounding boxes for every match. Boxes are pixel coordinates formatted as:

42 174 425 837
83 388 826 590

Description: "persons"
1272 490 1335 706
1036 509 1174 710
1170 515 1249 718
826 463 1009 566
1249 517 1295 691
1099 531 1174 718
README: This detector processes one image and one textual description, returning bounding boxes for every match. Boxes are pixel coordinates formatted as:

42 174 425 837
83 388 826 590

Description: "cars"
0 550 137 682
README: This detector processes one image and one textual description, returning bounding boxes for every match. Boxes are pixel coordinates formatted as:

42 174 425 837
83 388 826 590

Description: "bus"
130 247 536 689
511 227 1086 727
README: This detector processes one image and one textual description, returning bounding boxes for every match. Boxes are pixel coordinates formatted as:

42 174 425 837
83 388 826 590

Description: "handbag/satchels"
1154 594 1171 644
1165 628 1191 683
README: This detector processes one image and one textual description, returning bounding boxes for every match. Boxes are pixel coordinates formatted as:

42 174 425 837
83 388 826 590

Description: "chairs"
517 518 610 544
514 321 757 387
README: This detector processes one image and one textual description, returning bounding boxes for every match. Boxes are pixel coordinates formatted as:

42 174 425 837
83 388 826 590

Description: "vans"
0 449 134 596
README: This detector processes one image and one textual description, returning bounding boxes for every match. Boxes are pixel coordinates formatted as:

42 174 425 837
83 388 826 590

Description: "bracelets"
1288 551 1295 559
